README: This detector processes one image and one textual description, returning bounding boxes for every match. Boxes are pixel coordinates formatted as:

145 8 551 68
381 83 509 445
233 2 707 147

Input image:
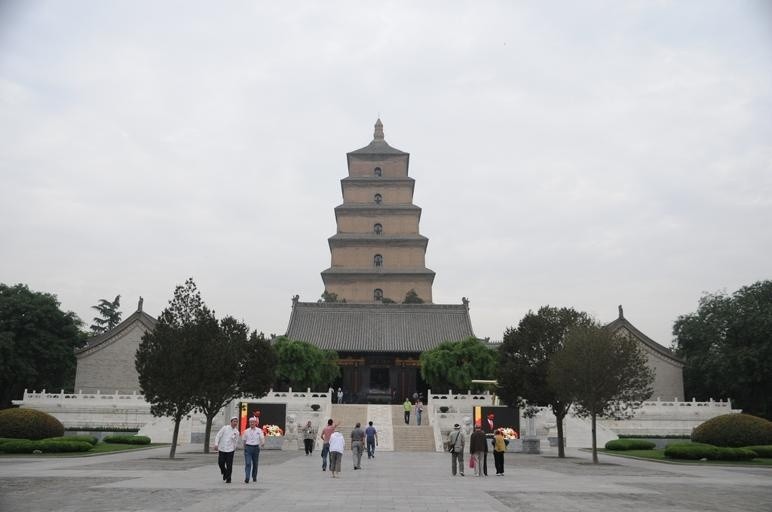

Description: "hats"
453 423 460 428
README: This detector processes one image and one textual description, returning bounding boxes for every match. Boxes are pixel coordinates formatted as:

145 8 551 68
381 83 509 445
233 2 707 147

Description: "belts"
352 439 363 441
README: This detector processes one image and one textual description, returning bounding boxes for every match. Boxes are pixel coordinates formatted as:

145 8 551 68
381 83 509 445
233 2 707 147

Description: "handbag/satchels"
469 453 475 469
448 444 454 453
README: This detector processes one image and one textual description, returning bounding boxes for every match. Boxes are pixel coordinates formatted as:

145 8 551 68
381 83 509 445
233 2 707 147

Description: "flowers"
260 423 284 436
495 426 519 440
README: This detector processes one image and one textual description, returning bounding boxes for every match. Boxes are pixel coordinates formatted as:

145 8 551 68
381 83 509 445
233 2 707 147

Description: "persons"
303 421 314 455
214 416 239 483
470 425 485 477
329 431 345 479
337 388 343 404
375 167 381 175
484 451 489 477
350 423 364 470
374 292 382 301
403 398 412 425
492 431 509 477
365 421 379 459
242 418 265 484
484 413 496 434
374 227 382 235
321 419 341 473
447 424 465 477
374 257 382 267
414 398 423 425
374 197 381 203
